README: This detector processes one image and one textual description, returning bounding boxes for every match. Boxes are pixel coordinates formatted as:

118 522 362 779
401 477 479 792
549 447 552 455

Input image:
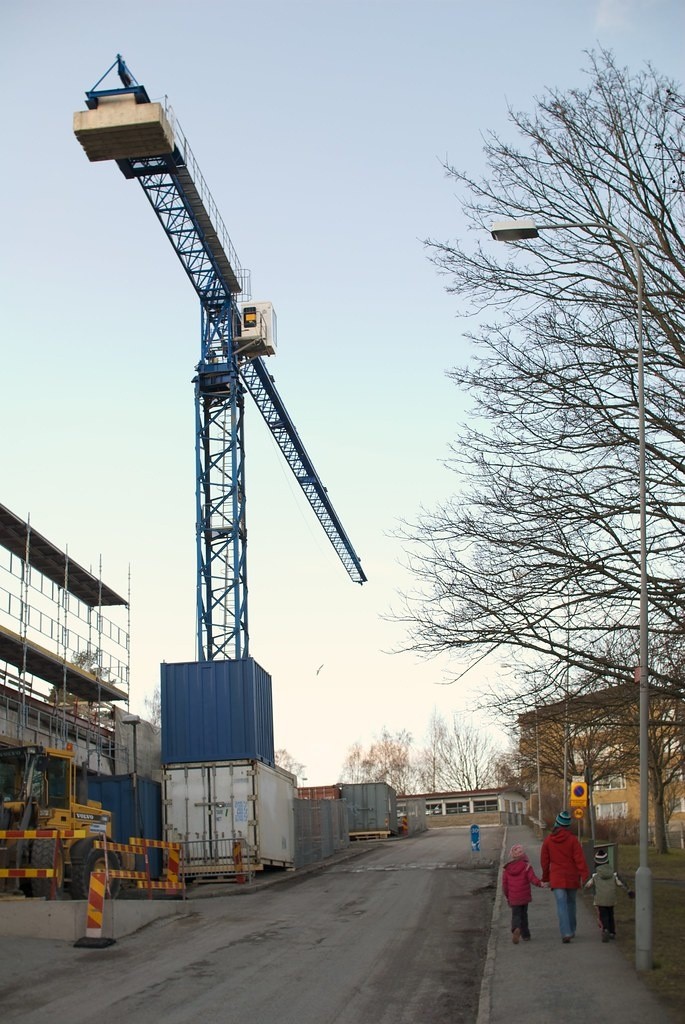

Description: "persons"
502 811 636 944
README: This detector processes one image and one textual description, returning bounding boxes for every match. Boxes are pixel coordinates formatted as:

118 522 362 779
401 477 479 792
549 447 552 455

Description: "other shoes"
525 936 530 941
602 929 609 942
608 933 614 938
512 927 521 944
562 936 570 943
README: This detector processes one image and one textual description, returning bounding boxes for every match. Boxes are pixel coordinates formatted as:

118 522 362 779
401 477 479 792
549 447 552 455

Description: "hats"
554 811 571 829
594 849 609 865
510 844 526 859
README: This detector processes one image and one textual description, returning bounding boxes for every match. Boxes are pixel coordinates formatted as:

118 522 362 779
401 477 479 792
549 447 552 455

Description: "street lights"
490 215 655 976
515 569 570 810
500 661 545 842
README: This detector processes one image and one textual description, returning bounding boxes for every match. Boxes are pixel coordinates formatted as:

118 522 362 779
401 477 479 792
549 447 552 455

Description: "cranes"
72 54 369 661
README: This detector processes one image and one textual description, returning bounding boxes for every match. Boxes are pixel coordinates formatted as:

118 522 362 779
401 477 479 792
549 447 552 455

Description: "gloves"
627 890 636 899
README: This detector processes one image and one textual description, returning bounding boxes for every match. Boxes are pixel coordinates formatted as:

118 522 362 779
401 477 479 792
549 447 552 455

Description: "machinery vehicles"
0 742 123 899
